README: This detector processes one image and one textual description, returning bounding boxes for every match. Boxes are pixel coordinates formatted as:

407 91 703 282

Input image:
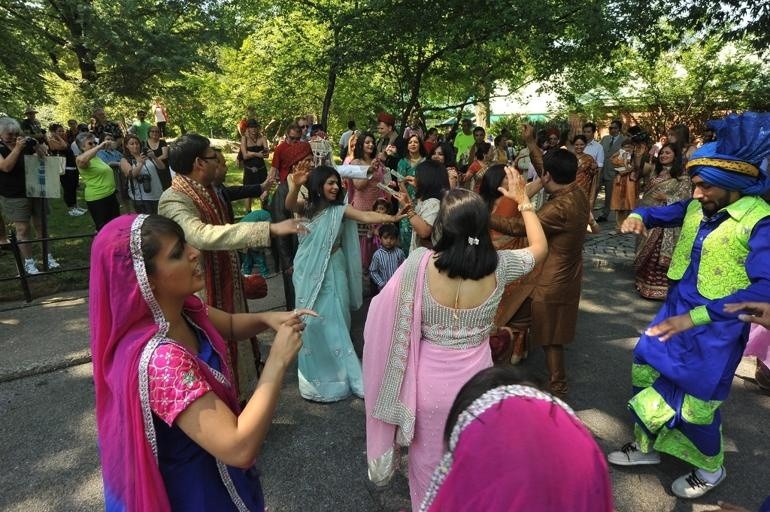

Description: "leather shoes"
595 216 607 222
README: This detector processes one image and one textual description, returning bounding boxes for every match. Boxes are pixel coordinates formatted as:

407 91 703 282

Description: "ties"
609 138 614 146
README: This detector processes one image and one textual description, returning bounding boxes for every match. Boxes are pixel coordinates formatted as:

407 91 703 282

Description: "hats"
24 107 39 114
246 119 260 128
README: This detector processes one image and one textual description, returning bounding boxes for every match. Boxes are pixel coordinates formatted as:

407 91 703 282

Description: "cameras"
142 146 148 155
111 142 117 149
24 136 37 146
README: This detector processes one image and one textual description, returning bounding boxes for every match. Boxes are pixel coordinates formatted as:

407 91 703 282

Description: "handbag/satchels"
24 143 67 198
237 149 247 160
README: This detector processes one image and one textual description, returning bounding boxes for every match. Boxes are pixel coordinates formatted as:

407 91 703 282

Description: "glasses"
200 156 220 163
289 136 299 140
301 125 309 129
88 142 97 145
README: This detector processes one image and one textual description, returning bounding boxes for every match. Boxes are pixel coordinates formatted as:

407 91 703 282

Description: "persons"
0 98 770 510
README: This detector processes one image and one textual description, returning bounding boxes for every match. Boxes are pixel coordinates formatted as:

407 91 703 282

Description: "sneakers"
671 465 727 498
24 258 39 274
68 207 88 216
607 441 661 466
48 254 60 269
